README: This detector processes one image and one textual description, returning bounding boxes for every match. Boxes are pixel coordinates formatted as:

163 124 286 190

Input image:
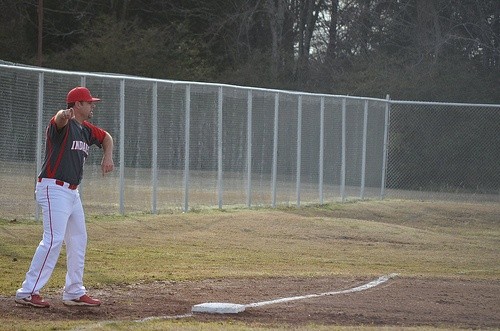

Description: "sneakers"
63 292 101 306
15 294 49 308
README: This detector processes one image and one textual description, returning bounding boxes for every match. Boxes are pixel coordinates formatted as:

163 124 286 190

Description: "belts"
37 178 77 190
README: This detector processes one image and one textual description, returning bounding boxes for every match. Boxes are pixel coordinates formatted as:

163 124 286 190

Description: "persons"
15 87 114 307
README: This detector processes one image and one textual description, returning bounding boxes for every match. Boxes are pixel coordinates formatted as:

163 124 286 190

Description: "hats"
66 87 102 104
